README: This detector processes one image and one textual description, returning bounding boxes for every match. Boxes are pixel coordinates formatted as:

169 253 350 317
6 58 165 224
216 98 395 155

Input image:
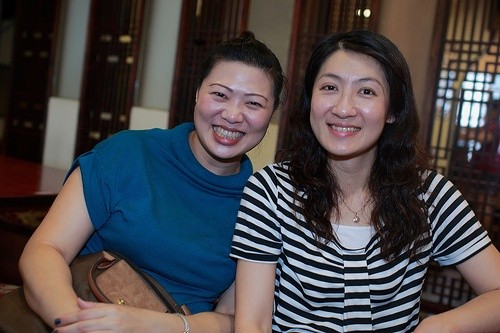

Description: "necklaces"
336 191 372 223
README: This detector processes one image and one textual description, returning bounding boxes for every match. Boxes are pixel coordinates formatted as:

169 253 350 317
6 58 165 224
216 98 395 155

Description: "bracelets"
174 313 190 333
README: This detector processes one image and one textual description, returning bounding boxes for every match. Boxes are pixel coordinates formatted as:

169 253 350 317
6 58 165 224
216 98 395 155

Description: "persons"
19 32 283 333
231 30 500 333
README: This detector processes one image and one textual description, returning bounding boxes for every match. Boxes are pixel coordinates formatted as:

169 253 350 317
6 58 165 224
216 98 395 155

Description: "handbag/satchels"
0 251 190 331
